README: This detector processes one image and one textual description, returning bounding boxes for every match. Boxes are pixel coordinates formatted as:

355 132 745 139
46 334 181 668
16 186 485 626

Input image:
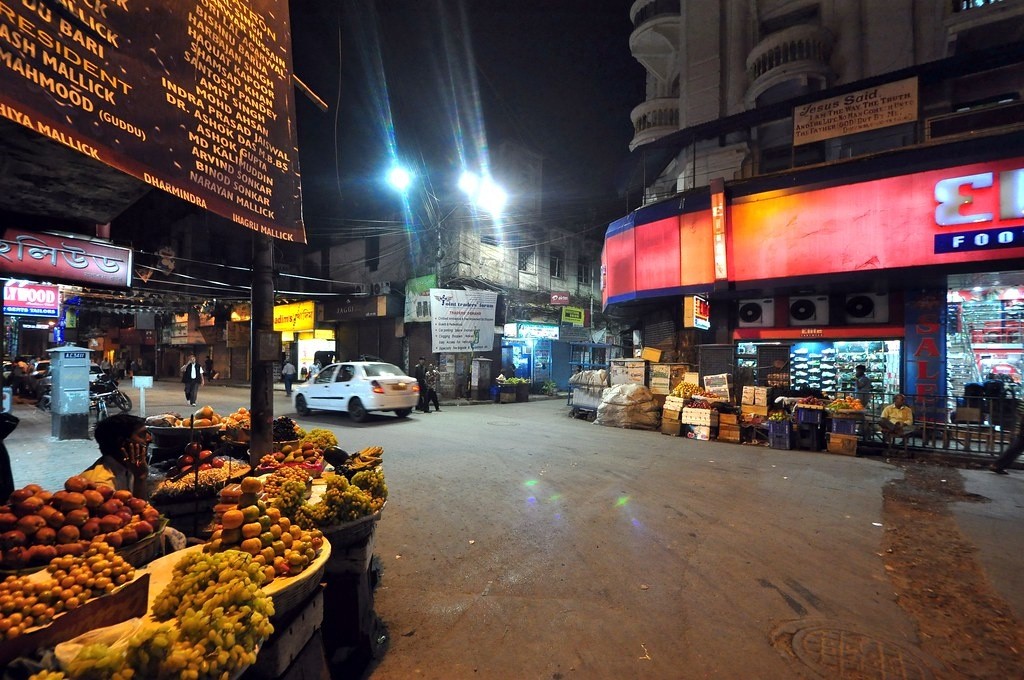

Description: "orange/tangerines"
831 396 863 409
203 477 322 582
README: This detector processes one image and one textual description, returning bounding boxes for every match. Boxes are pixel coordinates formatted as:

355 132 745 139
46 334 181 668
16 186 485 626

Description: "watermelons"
323 446 349 466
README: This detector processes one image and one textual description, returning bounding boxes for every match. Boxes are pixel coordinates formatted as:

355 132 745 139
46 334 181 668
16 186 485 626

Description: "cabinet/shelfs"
884 420 996 457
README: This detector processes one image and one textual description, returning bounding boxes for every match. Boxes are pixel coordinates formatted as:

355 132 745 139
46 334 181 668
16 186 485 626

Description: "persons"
501 364 516 379
426 364 441 412
80 414 211 543
575 365 582 373
204 356 213 382
11 356 41 396
332 358 352 370
181 355 204 406
879 394 913 444
415 357 433 413
100 358 149 380
855 365 872 407
282 360 296 397
308 358 322 380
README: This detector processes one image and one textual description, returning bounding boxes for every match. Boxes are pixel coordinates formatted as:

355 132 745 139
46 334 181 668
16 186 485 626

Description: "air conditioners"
373 281 392 295
738 299 774 327
354 283 372 296
845 291 890 324
789 294 830 326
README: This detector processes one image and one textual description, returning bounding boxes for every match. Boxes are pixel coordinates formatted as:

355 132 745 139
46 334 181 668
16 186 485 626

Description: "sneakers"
193 403 196 406
186 400 190 405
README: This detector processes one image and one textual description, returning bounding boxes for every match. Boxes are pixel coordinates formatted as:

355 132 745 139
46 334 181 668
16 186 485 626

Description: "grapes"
259 454 388 531
272 416 299 441
26 551 277 680
298 427 338 449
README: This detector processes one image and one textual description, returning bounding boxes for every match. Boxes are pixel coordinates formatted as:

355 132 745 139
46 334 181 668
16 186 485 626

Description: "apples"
165 442 224 476
672 381 723 411
797 396 823 405
0 476 159 572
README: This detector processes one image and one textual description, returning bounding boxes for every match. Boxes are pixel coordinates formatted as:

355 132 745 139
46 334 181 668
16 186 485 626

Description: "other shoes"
988 467 1009 474
416 408 442 413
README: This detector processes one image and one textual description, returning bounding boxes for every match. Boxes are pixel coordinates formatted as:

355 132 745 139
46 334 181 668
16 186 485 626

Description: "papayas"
168 406 226 426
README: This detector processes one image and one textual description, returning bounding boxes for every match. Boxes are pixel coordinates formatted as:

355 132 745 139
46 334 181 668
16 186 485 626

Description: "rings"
136 455 141 459
124 458 129 460
131 461 135 463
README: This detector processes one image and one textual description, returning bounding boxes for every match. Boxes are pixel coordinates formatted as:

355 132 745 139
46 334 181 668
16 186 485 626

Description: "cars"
28 363 110 407
289 360 421 422
18 360 52 399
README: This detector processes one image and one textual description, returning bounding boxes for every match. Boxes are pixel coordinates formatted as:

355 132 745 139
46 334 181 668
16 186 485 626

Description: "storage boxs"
661 375 865 456
250 591 323 675
495 383 530 403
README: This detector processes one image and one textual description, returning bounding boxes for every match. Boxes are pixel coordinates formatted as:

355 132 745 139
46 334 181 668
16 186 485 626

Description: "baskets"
0 518 168 582
139 531 330 620
145 418 300 453
321 500 387 546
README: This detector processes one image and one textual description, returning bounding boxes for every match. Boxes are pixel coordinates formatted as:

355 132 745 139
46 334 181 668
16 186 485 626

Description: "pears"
768 412 783 421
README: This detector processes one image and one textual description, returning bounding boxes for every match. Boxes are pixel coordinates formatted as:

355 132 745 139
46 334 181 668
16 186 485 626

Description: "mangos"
220 407 250 429
274 442 323 463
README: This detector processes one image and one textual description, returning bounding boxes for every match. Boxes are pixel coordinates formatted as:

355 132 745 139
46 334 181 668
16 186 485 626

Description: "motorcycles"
42 371 133 414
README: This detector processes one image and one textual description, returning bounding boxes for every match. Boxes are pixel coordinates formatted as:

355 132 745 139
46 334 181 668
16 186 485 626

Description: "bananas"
506 377 526 384
346 446 384 468
